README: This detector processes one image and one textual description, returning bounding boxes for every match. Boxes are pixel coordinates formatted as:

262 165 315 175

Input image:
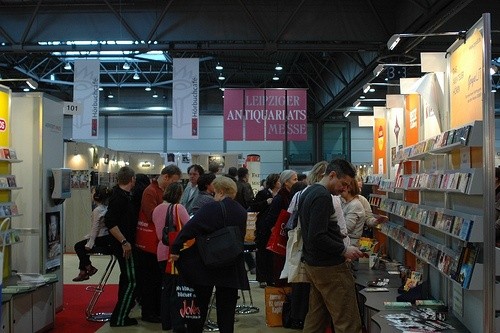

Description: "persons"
72 185 111 281
168 175 248 333
251 173 282 288
298 174 307 181
222 167 238 182
283 181 307 328
133 164 182 322
187 172 216 217
152 183 190 330
279 158 351 333
354 175 389 227
258 179 266 191
234 167 254 212
339 178 365 262
177 163 204 215
104 167 139 327
131 173 151 203
297 158 366 333
265 170 298 284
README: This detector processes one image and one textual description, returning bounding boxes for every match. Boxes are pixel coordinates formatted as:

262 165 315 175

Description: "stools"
82 240 258 333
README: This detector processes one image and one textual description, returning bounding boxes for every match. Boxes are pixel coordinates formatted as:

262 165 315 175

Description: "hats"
280 170 297 185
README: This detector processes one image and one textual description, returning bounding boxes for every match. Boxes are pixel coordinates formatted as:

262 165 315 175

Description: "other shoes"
141 314 160 323
251 269 256 274
261 282 267 287
79 266 98 276
124 317 138 326
72 274 89 281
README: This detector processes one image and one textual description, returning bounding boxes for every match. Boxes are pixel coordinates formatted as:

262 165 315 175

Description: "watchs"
121 239 127 245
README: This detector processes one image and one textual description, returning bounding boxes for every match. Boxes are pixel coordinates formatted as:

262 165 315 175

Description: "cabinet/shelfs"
0 85 58 333
352 120 484 333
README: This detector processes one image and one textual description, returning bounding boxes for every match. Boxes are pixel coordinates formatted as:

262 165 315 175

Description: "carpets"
42 283 120 333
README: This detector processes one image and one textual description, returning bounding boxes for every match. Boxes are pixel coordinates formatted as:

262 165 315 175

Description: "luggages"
151 260 178 330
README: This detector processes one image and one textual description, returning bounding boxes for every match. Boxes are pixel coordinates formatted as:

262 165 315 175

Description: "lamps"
61 50 283 97
344 107 373 117
0 79 38 90
362 83 400 93
373 64 422 77
353 99 386 108
387 32 460 51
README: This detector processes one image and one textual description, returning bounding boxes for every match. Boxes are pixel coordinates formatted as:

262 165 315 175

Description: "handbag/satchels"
265 286 293 328
165 204 183 275
281 292 310 330
197 201 244 266
266 209 291 257
162 203 177 246
279 216 312 282
284 189 302 229
